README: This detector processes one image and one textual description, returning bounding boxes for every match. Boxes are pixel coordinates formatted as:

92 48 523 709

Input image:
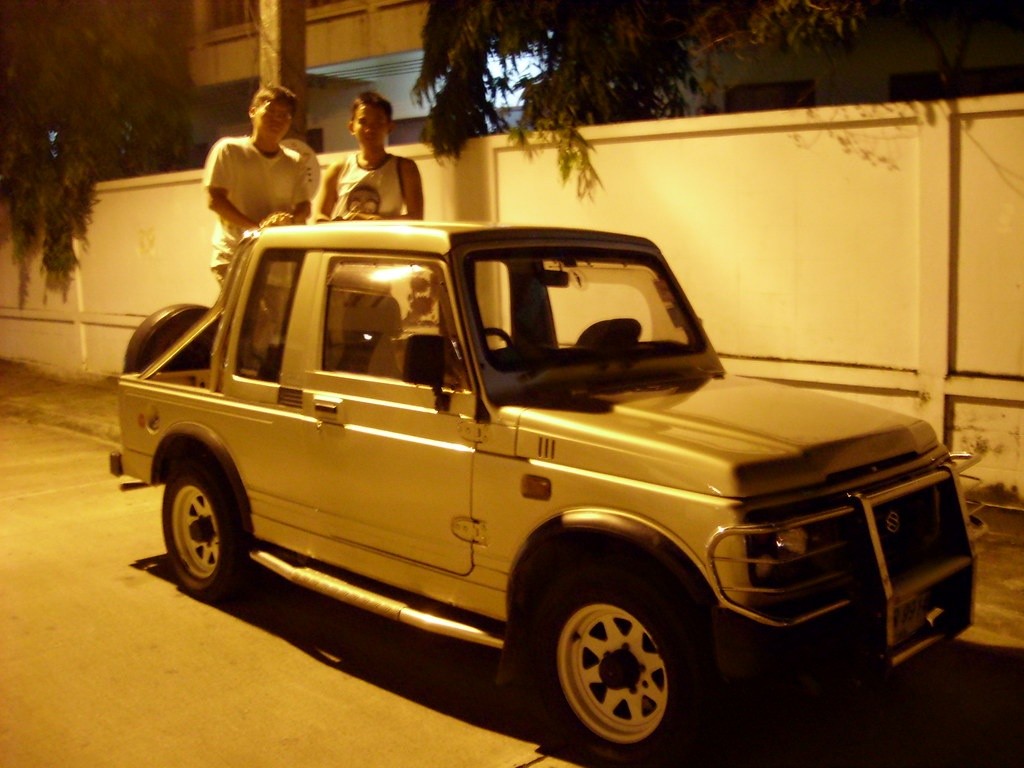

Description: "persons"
202 82 311 373
310 90 425 225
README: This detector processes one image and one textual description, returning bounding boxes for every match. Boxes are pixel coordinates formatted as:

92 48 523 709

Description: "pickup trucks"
109 219 990 767
